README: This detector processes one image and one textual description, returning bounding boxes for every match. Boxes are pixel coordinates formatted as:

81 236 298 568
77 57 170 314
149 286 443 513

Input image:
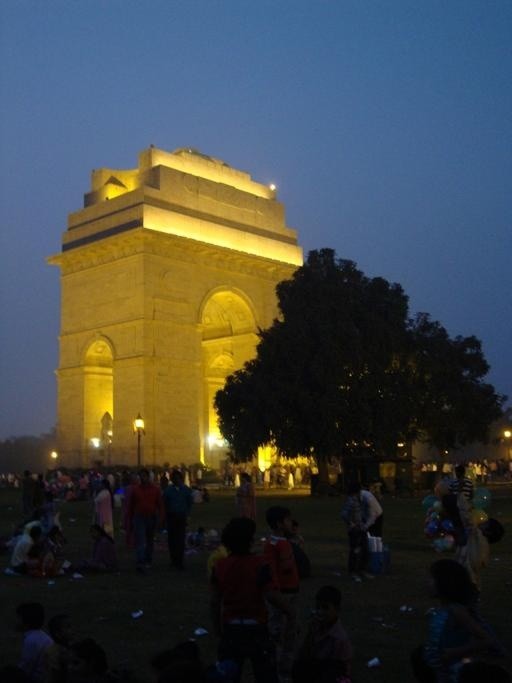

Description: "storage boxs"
350 550 391 575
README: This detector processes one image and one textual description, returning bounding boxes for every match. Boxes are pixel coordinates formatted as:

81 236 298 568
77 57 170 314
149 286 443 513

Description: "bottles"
368 537 383 553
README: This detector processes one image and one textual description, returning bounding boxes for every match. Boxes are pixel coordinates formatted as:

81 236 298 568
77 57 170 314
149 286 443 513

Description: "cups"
367 657 381 668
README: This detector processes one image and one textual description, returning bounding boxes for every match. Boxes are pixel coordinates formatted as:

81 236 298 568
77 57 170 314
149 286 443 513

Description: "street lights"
133 410 145 469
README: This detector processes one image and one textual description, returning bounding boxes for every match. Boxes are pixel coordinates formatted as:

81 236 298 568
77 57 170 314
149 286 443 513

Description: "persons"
2 449 510 683
